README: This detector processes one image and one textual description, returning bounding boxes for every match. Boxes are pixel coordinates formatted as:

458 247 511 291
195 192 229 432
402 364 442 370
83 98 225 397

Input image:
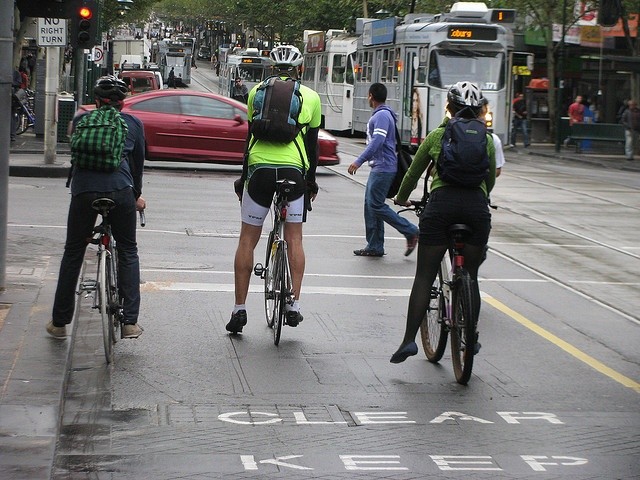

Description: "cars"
75 89 341 166
197 45 211 61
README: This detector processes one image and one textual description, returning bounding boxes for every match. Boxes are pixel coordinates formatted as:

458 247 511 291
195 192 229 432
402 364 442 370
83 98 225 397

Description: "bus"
149 38 196 85
218 46 272 98
300 29 358 135
350 2 517 154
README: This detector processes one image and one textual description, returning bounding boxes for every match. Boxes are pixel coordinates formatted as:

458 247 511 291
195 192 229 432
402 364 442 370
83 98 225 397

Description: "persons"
582 104 600 150
479 96 502 176
167 67 177 89
348 83 419 257
621 100 640 160
410 89 423 143
215 61 220 76
389 81 496 364
45 75 146 340
210 54 218 67
232 77 249 104
430 64 447 78
225 44 322 331
143 56 149 70
563 94 584 149
192 56 197 69
20 52 35 88
510 94 531 147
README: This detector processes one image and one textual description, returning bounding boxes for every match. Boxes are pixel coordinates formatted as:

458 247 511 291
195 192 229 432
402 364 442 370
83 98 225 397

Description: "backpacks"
438 105 490 186
249 76 310 144
66 105 128 188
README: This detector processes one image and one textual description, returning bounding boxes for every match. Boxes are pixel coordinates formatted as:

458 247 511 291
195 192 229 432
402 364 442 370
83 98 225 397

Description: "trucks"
109 40 144 76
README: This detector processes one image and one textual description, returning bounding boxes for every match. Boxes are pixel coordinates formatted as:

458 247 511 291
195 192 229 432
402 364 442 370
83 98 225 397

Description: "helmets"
93 74 127 100
268 45 304 67
447 80 486 108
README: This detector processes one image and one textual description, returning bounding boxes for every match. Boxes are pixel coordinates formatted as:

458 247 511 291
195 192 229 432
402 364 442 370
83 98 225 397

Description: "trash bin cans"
58 91 77 144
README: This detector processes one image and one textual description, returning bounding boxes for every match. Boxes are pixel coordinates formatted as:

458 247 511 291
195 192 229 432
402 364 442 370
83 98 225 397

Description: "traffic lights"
71 0 99 50
14 0 71 19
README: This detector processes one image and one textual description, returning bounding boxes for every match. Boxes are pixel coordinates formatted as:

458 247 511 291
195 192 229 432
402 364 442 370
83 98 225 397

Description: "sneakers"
286 310 304 326
225 309 247 334
474 341 482 355
45 320 67 340
121 323 144 339
389 342 416 364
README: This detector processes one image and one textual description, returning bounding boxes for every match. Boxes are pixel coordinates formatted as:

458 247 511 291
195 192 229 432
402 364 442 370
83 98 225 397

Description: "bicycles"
254 178 318 345
68 190 145 364
394 199 480 384
11 90 34 135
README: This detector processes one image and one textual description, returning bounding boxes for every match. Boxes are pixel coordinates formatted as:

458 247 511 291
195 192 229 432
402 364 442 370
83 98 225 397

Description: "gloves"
309 182 318 202
234 179 244 199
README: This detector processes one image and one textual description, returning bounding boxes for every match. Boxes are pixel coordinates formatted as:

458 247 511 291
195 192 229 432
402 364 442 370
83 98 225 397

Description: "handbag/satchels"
371 108 417 198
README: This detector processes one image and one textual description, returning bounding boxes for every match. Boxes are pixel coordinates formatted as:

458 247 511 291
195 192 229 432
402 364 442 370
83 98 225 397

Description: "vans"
119 71 162 95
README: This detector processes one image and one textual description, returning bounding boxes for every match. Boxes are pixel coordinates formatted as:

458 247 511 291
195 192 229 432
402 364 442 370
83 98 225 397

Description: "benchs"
570 122 626 154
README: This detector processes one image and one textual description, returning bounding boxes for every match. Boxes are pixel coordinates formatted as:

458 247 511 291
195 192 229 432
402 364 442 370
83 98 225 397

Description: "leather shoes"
405 228 421 257
353 245 384 257
